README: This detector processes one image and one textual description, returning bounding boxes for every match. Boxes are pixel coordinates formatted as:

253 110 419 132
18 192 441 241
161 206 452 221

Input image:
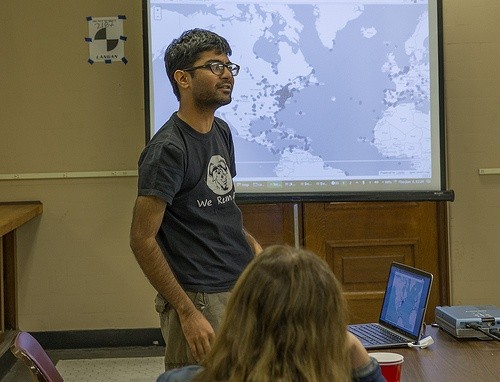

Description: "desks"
0 201 42 357
366 325 500 382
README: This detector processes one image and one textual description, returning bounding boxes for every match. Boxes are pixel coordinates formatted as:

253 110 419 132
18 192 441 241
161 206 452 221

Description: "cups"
369 352 404 382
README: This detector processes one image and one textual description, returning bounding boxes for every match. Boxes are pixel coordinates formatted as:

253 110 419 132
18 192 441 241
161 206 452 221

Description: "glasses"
182 59 241 77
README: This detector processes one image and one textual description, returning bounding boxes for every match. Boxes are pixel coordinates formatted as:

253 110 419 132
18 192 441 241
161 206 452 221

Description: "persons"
156 244 387 382
129 28 264 382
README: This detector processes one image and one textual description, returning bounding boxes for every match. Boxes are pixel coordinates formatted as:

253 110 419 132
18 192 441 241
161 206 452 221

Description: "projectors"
435 305 500 341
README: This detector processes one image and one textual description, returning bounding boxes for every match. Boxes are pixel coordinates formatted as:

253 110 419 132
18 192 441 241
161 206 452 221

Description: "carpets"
54 356 165 382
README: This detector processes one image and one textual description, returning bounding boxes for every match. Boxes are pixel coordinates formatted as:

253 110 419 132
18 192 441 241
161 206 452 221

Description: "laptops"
344 261 433 350
